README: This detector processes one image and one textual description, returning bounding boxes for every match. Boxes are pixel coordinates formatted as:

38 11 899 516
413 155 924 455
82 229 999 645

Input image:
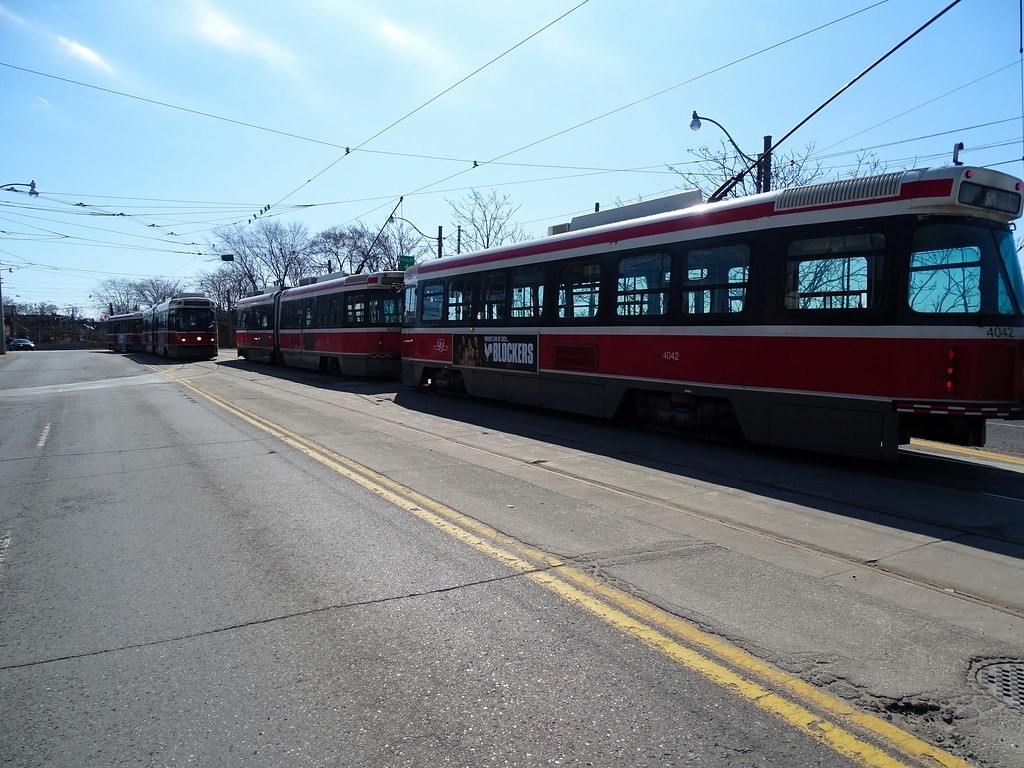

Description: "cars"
9 339 35 351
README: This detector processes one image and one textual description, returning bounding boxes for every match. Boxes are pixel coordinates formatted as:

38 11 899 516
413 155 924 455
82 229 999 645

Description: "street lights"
688 110 773 194
389 215 443 258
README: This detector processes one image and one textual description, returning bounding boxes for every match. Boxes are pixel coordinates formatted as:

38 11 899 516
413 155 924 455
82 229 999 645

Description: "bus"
104 293 219 362
402 141 1022 468
234 270 407 383
